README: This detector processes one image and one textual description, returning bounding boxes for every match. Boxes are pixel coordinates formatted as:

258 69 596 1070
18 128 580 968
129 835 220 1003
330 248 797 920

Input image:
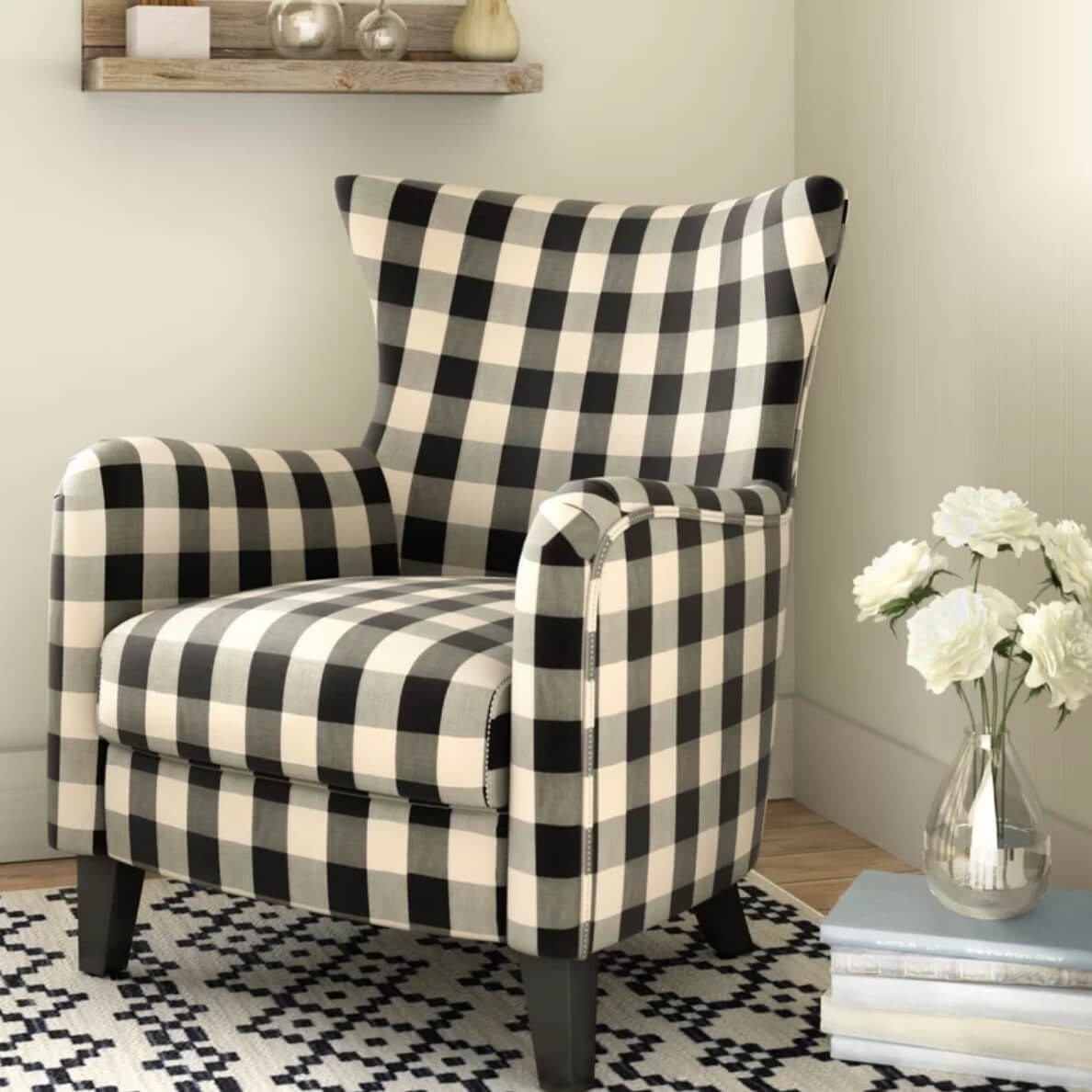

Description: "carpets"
0 869 1092 1092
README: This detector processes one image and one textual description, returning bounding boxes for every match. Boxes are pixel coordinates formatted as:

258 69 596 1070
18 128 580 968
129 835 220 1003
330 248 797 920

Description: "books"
819 868 1092 1092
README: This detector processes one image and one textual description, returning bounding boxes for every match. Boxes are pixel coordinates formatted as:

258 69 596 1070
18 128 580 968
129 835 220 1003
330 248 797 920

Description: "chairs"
42 173 851 1092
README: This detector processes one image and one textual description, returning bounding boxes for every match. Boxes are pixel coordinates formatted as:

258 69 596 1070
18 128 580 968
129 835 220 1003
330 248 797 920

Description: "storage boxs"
125 4 211 59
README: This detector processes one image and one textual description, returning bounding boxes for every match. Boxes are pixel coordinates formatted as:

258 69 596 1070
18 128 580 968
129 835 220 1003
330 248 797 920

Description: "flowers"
850 486 1092 851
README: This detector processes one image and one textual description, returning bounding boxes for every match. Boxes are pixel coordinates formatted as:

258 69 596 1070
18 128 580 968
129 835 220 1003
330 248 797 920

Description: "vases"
921 726 1054 920
